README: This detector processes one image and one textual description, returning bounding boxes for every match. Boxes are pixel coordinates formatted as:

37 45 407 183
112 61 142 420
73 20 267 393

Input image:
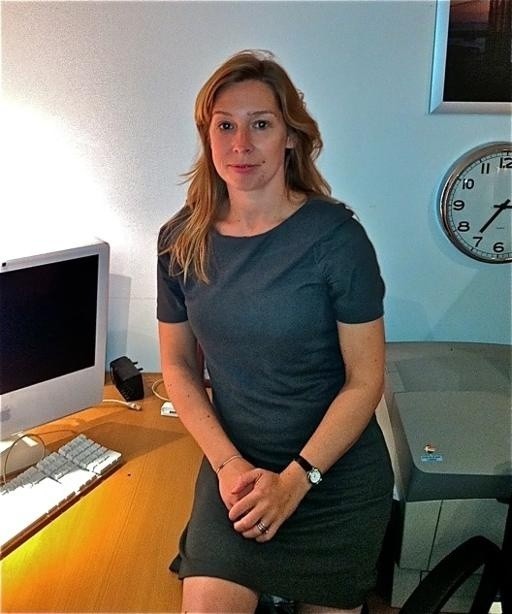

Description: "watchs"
293 454 324 487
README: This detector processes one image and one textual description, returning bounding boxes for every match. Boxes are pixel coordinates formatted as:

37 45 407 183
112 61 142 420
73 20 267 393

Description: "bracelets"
215 453 244 476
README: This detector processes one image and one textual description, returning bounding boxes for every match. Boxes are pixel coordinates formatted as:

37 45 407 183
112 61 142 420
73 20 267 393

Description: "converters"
109 355 145 401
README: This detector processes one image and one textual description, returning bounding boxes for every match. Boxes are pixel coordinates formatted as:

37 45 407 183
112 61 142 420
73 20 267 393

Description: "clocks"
433 144 512 263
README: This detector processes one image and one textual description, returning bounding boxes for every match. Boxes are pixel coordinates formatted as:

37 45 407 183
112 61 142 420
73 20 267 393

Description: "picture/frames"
429 1 512 113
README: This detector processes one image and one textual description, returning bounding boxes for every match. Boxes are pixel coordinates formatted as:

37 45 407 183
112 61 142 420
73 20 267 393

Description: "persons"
156 52 397 611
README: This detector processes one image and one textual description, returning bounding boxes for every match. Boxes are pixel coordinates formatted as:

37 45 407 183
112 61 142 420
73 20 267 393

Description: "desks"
1 370 207 613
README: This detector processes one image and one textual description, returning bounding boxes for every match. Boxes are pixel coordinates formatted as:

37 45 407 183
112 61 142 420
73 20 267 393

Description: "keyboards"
0 431 123 562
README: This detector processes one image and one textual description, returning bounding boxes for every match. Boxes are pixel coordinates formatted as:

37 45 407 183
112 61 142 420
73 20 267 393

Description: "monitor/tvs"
0 236 110 477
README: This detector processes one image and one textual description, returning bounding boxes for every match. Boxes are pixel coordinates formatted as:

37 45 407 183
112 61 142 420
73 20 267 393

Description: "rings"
257 520 267 533
